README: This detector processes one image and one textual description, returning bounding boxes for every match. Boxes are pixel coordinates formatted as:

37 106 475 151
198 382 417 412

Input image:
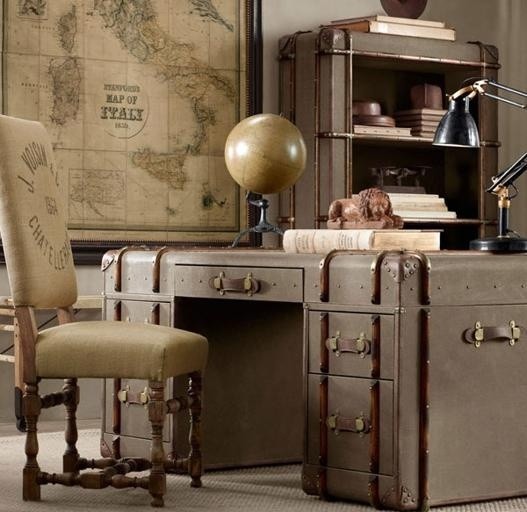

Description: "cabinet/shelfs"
100 252 300 473
300 256 526 511
278 30 502 248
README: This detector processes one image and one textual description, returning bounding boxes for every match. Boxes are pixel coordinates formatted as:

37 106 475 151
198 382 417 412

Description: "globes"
224 112 308 250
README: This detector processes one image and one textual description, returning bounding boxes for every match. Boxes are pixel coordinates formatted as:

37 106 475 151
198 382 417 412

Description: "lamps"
432 74 526 253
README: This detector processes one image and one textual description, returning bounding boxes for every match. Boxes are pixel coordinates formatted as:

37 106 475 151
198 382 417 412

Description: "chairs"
0 113 210 509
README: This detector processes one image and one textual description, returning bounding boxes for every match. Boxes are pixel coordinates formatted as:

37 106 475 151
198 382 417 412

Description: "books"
319 20 457 43
385 193 459 221
351 124 413 138
329 15 446 29
389 107 447 137
280 229 447 255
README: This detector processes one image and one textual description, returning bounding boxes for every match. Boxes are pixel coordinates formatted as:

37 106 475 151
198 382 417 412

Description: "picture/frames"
0 0 262 267
